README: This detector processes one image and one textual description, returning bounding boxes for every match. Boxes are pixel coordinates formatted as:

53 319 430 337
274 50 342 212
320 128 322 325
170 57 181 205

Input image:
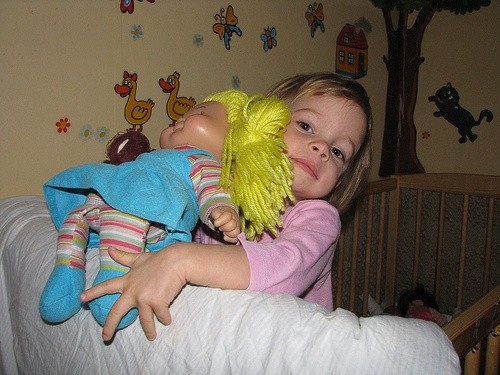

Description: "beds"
1 172 500 375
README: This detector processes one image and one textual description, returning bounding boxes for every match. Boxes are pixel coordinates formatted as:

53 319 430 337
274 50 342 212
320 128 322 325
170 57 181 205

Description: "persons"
38 88 296 329
80 72 373 341
